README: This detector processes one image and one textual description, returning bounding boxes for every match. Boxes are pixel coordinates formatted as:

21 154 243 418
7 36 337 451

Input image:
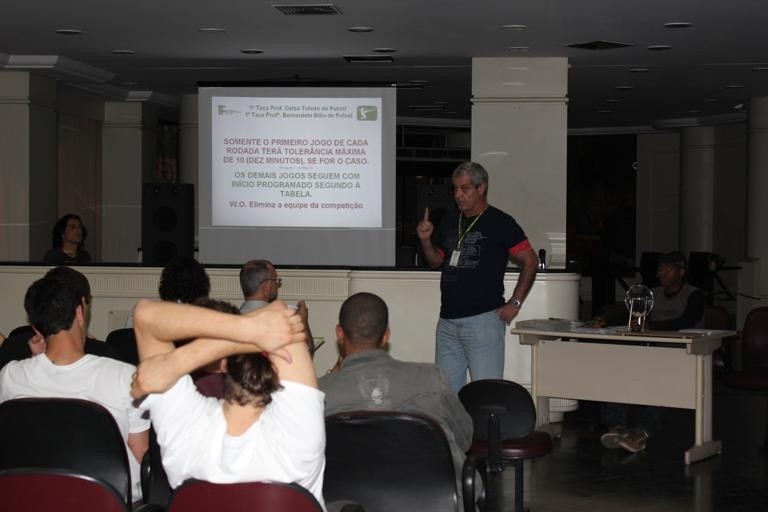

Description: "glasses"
261 276 282 287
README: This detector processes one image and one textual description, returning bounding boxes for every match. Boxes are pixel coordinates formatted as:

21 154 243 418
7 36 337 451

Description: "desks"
510 328 738 466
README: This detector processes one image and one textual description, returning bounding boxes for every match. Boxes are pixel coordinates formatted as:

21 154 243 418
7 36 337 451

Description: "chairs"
600 304 768 454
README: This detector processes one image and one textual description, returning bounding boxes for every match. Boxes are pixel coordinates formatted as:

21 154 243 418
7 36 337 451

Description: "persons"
2 279 145 498
43 267 96 341
2 324 46 361
130 294 329 508
36 213 94 264
415 161 538 393
236 259 281 319
156 256 210 306
598 252 707 455
187 291 241 399
316 293 486 512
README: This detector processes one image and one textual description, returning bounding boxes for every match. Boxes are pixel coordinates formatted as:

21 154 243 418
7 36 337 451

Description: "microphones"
538 249 550 273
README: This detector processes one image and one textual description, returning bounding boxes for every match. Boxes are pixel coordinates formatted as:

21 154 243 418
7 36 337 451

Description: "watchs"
507 296 522 311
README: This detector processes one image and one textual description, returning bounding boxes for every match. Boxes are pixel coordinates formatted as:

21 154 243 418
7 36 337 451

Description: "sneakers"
601 425 631 449
619 431 649 452
